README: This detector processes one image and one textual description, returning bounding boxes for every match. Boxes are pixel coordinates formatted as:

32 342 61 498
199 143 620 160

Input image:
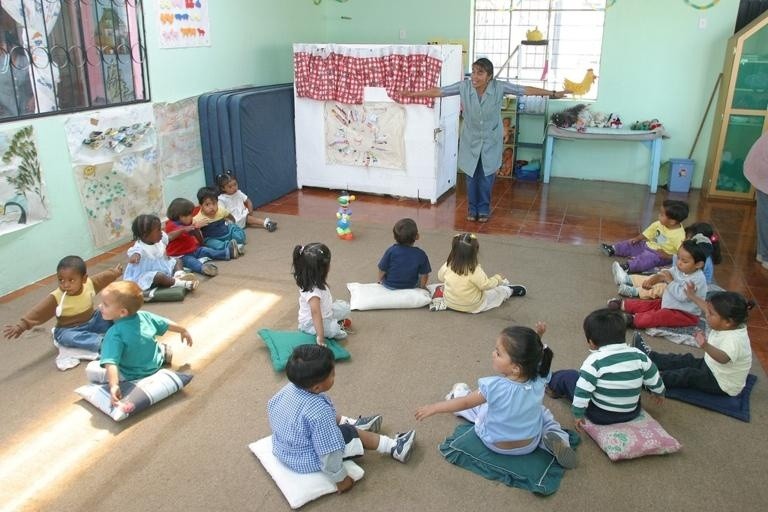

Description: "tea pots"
526 26 544 41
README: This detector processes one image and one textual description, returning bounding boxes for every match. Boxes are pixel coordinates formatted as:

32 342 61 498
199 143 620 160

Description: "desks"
542 120 673 195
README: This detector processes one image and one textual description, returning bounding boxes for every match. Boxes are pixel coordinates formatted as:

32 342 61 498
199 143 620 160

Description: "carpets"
0 206 767 511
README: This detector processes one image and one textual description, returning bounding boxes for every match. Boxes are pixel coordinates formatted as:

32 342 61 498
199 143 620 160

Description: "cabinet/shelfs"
700 8 768 203
290 43 462 207
493 93 517 179
512 94 549 183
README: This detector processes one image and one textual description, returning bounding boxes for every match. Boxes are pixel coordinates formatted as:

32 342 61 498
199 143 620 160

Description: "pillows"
581 412 683 462
426 283 448 312
51 334 107 364
651 367 759 425
244 430 367 511
255 324 350 374
343 279 431 314
139 282 187 303
71 368 194 427
436 419 580 495
648 316 709 348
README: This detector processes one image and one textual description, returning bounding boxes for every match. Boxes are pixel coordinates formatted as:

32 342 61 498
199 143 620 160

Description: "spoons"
55 290 67 318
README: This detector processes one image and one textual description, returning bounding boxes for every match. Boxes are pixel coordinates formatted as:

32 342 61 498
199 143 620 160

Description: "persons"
336 194 355 240
267 344 417 495
546 308 666 424
216 169 278 232
85 281 192 406
289 242 350 348
600 200 756 398
437 232 527 314
192 186 245 259
164 197 239 278
377 218 432 291
2 255 123 355
413 322 577 470
123 215 200 291
405 57 567 223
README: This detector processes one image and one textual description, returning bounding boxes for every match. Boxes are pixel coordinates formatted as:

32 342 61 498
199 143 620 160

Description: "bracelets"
553 91 556 96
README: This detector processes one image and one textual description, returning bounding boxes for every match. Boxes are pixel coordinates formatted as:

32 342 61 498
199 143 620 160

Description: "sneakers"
266 221 276 231
608 261 634 326
176 273 198 290
601 244 615 257
445 383 471 399
163 343 173 363
543 432 577 468
391 430 415 462
202 263 217 277
508 284 527 296
355 415 382 431
632 331 652 355
230 239 244 258
